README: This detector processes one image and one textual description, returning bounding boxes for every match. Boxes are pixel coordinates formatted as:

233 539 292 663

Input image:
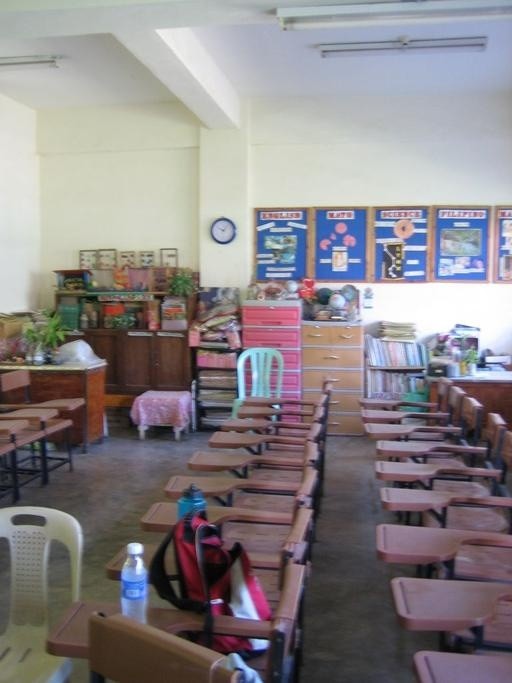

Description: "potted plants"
19 309 72 365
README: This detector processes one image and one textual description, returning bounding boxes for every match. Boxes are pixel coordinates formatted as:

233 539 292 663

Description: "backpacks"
148 507 274 657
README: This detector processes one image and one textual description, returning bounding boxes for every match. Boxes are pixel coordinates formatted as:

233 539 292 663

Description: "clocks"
210 217 235 244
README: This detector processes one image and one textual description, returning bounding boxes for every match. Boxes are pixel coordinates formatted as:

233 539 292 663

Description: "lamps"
1 63 57 72
321 45 487 63
280 9 512 30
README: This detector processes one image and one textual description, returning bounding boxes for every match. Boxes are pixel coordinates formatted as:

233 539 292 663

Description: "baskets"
196 350 236 368
198 391 237 405
204 409 234 425
199 371 237 388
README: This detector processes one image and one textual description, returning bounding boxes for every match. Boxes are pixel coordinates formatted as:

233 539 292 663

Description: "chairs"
0 409 60 486
1 371 85 472
0 506 83 671
0 419 31 506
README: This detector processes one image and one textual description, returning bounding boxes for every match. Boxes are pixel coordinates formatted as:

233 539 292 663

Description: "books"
376 321 417 341
364 369 425 401
364 336 421 367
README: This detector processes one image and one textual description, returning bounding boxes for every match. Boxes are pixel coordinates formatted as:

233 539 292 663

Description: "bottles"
176 482 207 527
80 314 88 328
121 543 146 626
90 311 98 328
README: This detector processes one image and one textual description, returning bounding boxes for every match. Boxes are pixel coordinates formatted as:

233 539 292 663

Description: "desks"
3 361 107 453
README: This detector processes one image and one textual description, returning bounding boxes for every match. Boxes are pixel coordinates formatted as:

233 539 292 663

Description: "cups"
467 363 476 376
447 364 460 378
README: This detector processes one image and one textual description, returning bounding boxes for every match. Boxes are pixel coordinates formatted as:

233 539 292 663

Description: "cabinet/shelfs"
66 328 116 407
302 325 364 437
240 300 302 422
112 330 189 411
192 345 242 433
366 339 427 412
430 370 512 426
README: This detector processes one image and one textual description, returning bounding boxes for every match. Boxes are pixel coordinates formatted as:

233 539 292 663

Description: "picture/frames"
250 208 512 285
78 245 178 271
